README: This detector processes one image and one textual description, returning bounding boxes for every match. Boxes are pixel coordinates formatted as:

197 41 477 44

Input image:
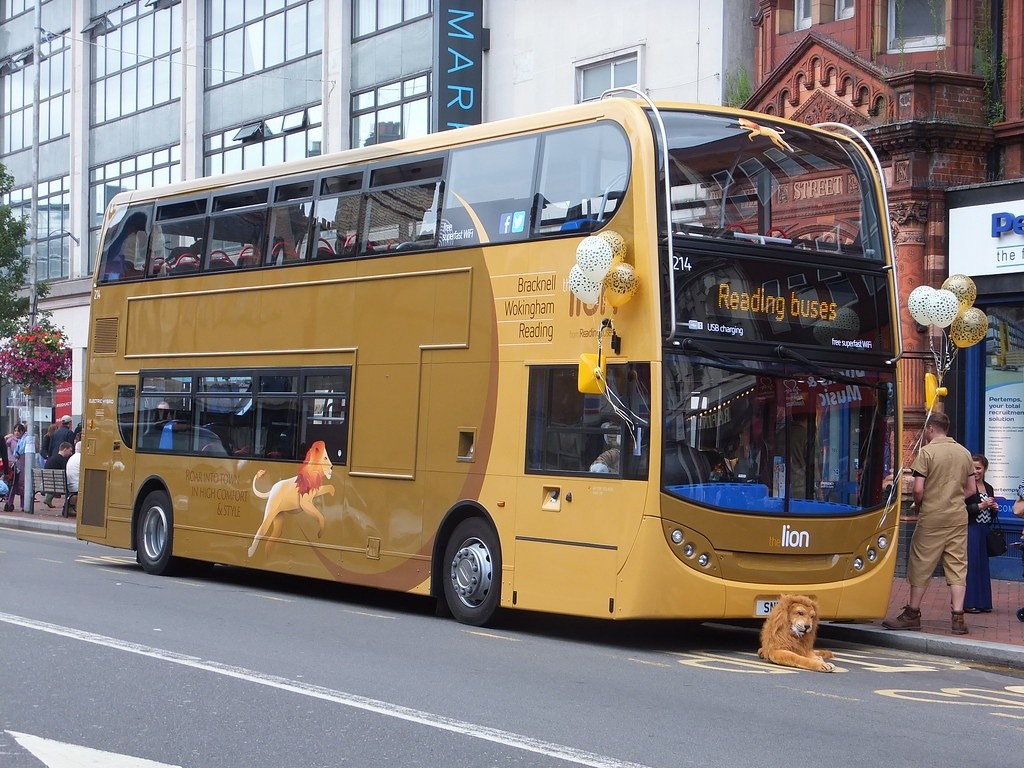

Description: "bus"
75 85 949 629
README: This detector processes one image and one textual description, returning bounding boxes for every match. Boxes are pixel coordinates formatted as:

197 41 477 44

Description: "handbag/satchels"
987 509 1008 557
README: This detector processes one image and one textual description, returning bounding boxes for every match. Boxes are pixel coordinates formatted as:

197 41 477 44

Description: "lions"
758 594 836 673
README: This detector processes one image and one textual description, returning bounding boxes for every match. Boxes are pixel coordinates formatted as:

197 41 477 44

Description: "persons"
882 413 977 636
0 414 82 515
778 400 824 501
964 454 999 613
1013 478 1024 518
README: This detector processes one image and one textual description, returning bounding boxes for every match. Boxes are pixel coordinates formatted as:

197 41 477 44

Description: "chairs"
153 232 432 277
765 227 789 239
141 417 349 464
724 224 749 241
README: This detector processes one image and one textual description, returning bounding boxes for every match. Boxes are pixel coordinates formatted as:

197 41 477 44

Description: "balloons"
941 274 977 309
577 236 612 283
926 289 958 329
569 263 602 305
951 307 988 348
603 263 641 308
597 230 627 262
908 286 936 326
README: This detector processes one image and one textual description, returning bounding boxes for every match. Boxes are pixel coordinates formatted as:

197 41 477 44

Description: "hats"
60 415 72 423
24 423 37 430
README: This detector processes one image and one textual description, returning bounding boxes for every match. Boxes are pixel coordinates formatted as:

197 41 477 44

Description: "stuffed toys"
588 422 622 474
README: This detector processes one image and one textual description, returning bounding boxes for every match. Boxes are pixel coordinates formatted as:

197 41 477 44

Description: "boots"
951 610 969 634
882 601 922 631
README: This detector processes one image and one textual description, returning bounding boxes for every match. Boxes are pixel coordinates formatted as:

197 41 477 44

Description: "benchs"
32 468 79 518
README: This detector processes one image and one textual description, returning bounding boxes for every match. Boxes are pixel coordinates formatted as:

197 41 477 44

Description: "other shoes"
44 500 55 508
963 606 990 613
